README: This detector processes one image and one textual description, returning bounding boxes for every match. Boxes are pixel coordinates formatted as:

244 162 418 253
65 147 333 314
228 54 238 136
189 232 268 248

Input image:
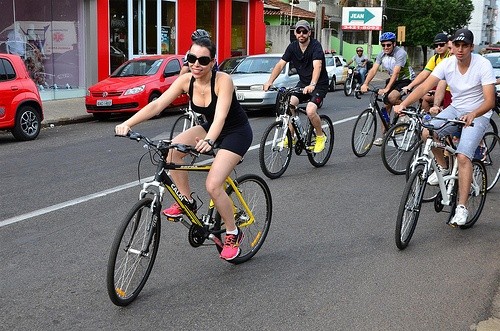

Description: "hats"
295 20 310 32
434 34 449 44
452 29 473 44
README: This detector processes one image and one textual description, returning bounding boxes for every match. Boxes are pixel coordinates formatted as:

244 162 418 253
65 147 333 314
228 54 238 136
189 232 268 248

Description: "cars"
85 54 191 122
0 54 45 141
214 56 248 75
225 53 323 116
483 43 500 98
323 48 350 92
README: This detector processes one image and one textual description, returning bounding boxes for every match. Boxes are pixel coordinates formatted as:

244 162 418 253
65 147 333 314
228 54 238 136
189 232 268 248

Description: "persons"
402 33 500 162
264 20 329 154
345 46 368 85
179 29 219 78
394 29 496 226
361 32 416 146
114 38 253 260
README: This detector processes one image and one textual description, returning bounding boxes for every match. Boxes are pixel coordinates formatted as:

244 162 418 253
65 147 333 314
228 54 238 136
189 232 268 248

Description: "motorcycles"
342 58 373 96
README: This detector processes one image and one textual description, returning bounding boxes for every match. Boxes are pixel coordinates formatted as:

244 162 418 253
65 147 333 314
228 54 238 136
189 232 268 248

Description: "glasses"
455 43 470 48
296 29 308 34
187 53 214 66
382 44 391 47
434 43 445 48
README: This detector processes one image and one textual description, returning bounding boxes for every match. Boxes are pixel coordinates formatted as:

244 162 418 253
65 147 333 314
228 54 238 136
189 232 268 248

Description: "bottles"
293 116 304 133
381 107 390 125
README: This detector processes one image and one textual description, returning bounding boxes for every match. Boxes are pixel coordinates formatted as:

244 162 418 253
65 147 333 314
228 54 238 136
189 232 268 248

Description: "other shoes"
374 138 384 144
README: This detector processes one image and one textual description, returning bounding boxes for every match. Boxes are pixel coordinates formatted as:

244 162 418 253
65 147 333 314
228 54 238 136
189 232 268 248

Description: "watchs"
310 81 317 86
204 139 215 148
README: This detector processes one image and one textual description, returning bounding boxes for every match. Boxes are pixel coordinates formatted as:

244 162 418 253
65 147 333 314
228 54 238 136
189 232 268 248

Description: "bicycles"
351 82 427 157
390 105 488 250
380 87 500 204
106 127 272 308
259 83 336 180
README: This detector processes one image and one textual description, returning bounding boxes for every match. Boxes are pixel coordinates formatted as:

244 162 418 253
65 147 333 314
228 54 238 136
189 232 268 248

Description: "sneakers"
314 133 327 153
451 204 468 225
278 136 296 147
162 196 197 218
428 165 450 185
219 226 245 260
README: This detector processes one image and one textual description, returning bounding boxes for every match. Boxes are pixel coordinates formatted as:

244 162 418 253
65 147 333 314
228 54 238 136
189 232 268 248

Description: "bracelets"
363 82 368 85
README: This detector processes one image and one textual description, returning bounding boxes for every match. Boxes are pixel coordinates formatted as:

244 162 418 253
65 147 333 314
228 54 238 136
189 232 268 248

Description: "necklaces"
196 70 212 95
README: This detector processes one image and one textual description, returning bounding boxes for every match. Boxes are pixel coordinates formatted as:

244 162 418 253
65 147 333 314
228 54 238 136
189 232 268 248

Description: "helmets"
191 29 209 41
379 32 396 42
356 46 363 51
